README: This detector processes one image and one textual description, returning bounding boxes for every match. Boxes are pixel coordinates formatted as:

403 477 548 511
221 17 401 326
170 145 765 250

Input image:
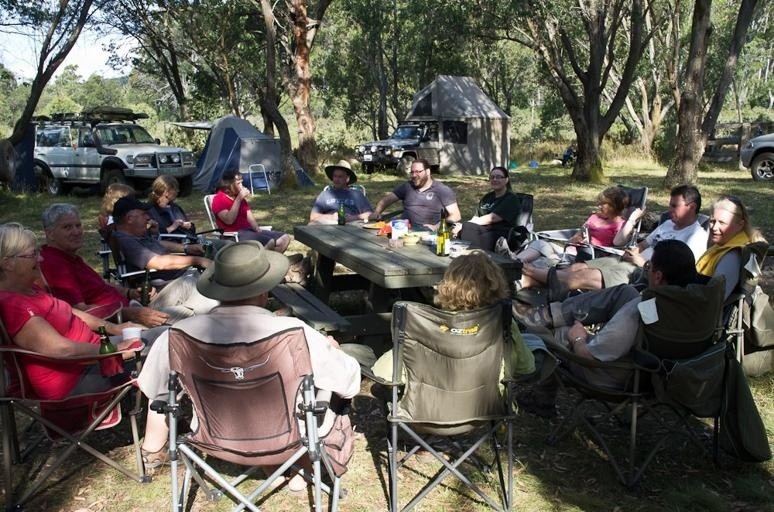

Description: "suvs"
356 117 441 176
32 105 197 198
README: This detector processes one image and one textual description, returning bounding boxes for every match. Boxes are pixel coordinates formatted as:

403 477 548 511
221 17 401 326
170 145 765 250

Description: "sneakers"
516 390 556 418
416 451 432 464
272 254 311 316
495 236 571 330
141 438 184 468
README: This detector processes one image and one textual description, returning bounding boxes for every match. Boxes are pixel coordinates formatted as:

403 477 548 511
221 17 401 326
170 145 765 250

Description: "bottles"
98 323 114 354
628 229 640 255
435 207 452 257
337 201 346 226
140 267 152 307
239 184 252 199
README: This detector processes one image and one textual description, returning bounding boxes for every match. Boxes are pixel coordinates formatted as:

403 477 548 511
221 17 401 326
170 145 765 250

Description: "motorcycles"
560 143 577 169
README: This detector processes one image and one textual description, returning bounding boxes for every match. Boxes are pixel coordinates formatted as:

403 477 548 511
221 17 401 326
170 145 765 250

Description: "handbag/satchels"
460 221 515 251
661 341 726 418
173 221 195 242
720 343 771 464
635 211 658 232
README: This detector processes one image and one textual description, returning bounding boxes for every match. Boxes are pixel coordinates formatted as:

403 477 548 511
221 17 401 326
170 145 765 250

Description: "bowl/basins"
399 236 420 246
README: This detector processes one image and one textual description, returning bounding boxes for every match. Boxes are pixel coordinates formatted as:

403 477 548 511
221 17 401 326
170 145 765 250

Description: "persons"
371 252 535 436
517 186 644 268
693 195 755 303
309 160 519 253
494 183 708 292
511 238 697 393
0 170 362 491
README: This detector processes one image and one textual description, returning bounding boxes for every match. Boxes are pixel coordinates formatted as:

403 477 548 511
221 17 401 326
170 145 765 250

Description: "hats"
325 160 356 184
113 196 153 216
197 240 289 302
517 333 560 386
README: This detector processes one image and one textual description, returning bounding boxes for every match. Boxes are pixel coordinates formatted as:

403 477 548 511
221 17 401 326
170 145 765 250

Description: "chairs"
95 193 274 288
1 300 150 512
508 189 534 253
527 273 734 489
341 299 554 511
536 186 648 263
722 240 771 364
658 209 711 230
149 326 350 511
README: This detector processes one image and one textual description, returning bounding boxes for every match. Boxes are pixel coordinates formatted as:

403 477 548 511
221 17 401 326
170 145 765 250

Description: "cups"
122 328 143 343
572 293 592 323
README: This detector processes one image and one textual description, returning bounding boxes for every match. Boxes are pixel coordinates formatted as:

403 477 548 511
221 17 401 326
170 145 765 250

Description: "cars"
740 130 774 183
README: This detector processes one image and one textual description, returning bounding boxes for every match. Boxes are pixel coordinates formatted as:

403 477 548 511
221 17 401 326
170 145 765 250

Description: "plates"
363 224 382 229
447 250 491 259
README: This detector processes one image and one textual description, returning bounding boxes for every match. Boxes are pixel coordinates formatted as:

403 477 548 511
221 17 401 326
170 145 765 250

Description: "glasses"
8 250 40 258
643 261 650 271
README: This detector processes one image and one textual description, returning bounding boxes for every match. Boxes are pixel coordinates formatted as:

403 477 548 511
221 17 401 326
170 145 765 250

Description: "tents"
188 117 315 192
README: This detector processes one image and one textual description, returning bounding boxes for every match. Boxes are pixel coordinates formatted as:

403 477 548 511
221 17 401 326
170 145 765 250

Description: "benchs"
269 282 350 335
